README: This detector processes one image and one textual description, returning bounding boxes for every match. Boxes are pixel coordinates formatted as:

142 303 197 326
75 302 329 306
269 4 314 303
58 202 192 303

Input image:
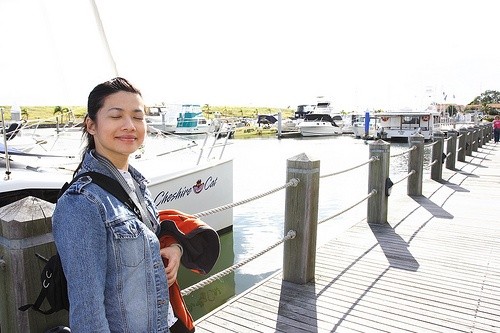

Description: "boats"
0 100 278 236
282 102 493 142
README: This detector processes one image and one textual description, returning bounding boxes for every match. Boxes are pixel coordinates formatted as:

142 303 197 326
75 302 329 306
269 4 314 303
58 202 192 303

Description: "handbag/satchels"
19 253 72 315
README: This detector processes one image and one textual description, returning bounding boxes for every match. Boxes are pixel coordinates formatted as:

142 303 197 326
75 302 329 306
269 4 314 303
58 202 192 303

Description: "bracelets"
171 243 183 256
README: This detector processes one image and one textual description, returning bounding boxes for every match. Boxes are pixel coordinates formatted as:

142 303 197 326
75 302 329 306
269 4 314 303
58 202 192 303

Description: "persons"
491 115 500 144
51 77 183 333
